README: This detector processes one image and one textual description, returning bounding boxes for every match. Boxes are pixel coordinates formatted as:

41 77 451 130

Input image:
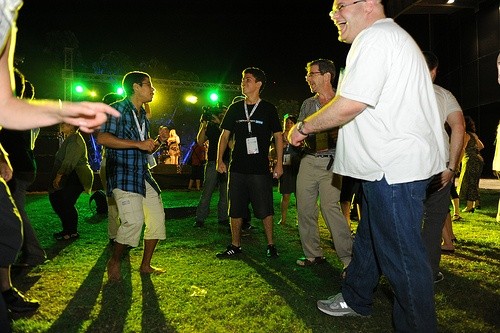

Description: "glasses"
304 71 327 78
329 0 366 18
137 81 152 88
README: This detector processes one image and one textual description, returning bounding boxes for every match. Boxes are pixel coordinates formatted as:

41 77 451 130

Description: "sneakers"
266 243 279 258
216 245 242 258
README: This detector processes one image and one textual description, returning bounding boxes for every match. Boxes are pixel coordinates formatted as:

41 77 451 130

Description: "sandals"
296 256 326 266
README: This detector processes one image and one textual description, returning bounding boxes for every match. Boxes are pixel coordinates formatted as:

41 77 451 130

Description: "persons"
99 71 167 281
268 115 302 227
147 96 251 236
216 68 284 259
287 0 449 333
418 49 484 283
295 59 354 279
340 175 363 230
0 0 124 333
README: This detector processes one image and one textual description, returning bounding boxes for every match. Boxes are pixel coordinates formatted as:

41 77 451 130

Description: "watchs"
297 120 309 136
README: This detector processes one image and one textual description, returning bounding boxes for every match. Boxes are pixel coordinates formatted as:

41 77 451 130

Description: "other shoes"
53 231 79 240
2 286 41 311
473 204 480 210
193 220 204 228
452 212 460 221
462 207 474 213
317 292 371 318
218 220 230 227
439 239 459 254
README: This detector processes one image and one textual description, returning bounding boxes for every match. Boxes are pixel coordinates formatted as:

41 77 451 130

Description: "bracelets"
448 167 455 173
58 98 63 124
